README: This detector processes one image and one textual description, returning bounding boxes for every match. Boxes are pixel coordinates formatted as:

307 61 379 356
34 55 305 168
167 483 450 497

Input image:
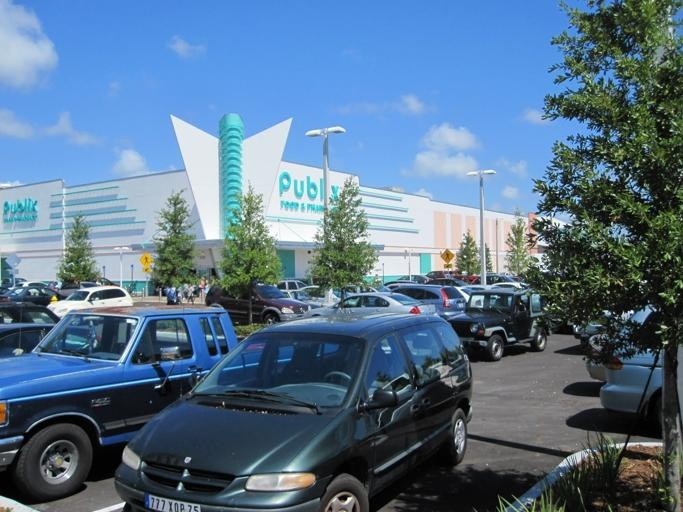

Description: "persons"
155 277 208 305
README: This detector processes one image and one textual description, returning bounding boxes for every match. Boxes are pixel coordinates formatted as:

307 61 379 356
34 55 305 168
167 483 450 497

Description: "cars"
549 302 665 431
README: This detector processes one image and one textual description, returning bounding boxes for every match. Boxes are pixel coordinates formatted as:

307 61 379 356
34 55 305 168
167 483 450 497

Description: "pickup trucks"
0 299 336 501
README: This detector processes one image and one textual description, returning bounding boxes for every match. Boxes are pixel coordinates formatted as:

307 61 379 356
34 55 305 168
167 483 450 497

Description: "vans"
109 304 479 512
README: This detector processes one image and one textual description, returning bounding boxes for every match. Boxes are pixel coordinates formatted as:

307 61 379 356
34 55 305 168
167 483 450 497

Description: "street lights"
405 248 415 282
299 125 348 305
113 246 129 291
463 168 497 284
